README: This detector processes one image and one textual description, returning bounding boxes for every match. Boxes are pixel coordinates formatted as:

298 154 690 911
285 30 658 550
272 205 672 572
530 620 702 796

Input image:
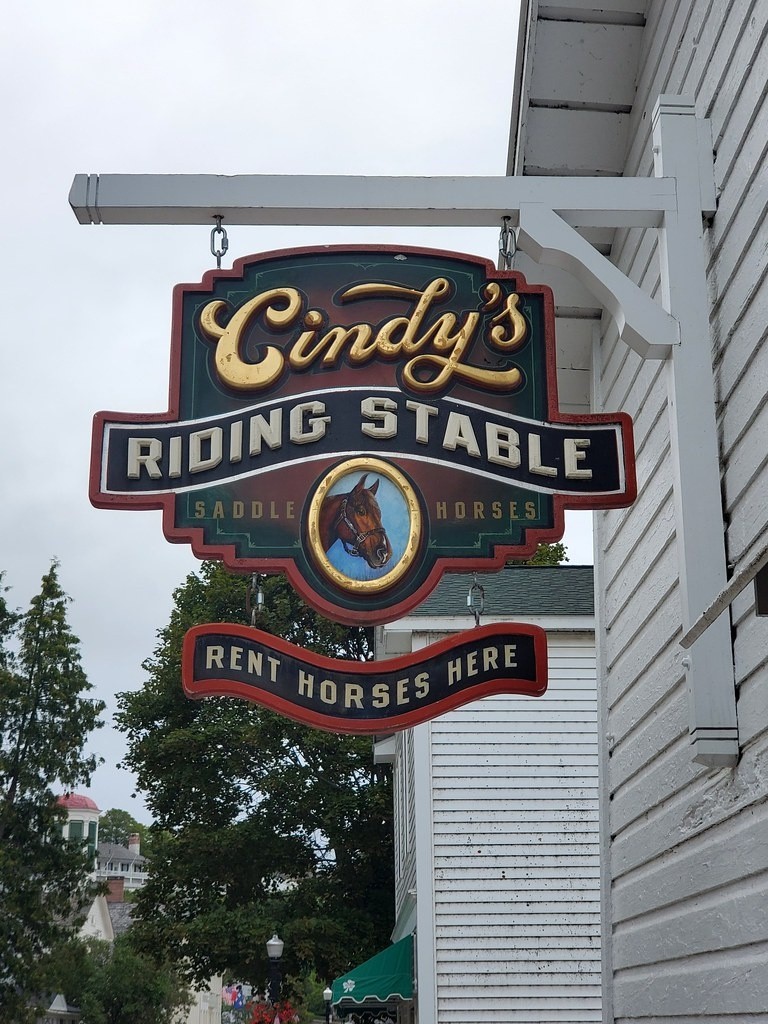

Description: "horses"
320 472 392 570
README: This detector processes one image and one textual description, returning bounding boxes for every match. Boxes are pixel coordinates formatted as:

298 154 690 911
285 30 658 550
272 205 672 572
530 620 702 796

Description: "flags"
222 986 243 1009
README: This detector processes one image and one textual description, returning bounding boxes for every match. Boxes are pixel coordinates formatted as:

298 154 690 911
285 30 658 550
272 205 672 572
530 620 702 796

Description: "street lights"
266 934 285 1001
323 987 333 1024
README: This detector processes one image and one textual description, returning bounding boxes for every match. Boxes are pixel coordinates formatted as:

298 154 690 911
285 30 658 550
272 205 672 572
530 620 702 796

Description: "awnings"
331 933 415 1017
